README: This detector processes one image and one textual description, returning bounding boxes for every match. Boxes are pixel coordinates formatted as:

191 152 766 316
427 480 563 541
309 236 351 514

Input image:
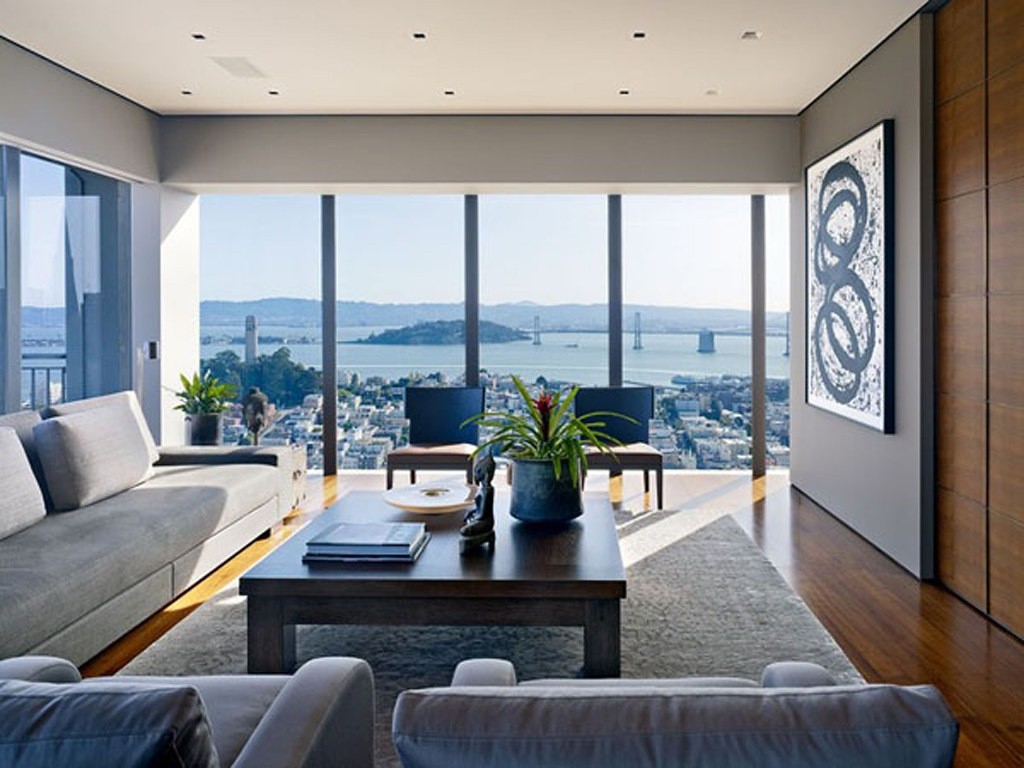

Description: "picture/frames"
803 117 896 433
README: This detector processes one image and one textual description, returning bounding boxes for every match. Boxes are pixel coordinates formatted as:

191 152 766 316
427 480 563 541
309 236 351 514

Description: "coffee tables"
238 489 629 678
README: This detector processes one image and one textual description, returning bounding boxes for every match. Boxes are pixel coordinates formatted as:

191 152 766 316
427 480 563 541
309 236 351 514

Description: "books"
303 521 431 562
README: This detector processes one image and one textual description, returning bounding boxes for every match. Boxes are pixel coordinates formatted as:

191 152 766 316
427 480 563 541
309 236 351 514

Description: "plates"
383 482 480 515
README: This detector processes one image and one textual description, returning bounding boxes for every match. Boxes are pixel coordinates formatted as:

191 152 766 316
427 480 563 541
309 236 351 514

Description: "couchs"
393 656 960 768
0 649 376 768
0 390 284 668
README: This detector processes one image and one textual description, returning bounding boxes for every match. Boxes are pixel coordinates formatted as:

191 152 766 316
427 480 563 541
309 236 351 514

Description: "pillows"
0 427 47 540
32 403 154 508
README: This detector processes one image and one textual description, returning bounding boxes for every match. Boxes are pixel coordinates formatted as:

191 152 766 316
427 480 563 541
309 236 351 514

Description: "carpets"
115 505 871 688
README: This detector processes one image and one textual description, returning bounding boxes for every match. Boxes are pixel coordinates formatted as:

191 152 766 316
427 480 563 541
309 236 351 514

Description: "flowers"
462 374 598 485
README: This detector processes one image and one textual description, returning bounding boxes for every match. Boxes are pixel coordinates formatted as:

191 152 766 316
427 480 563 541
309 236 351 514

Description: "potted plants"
174 370 233 445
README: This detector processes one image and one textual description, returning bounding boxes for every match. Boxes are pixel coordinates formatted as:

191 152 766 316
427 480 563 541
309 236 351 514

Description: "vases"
509 453 584 523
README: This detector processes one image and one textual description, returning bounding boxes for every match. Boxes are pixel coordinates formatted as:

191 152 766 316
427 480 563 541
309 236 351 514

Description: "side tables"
155 442 296 516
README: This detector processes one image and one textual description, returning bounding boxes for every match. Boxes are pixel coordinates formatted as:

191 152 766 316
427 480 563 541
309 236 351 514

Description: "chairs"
385 388 487 488
569 384 663 510
